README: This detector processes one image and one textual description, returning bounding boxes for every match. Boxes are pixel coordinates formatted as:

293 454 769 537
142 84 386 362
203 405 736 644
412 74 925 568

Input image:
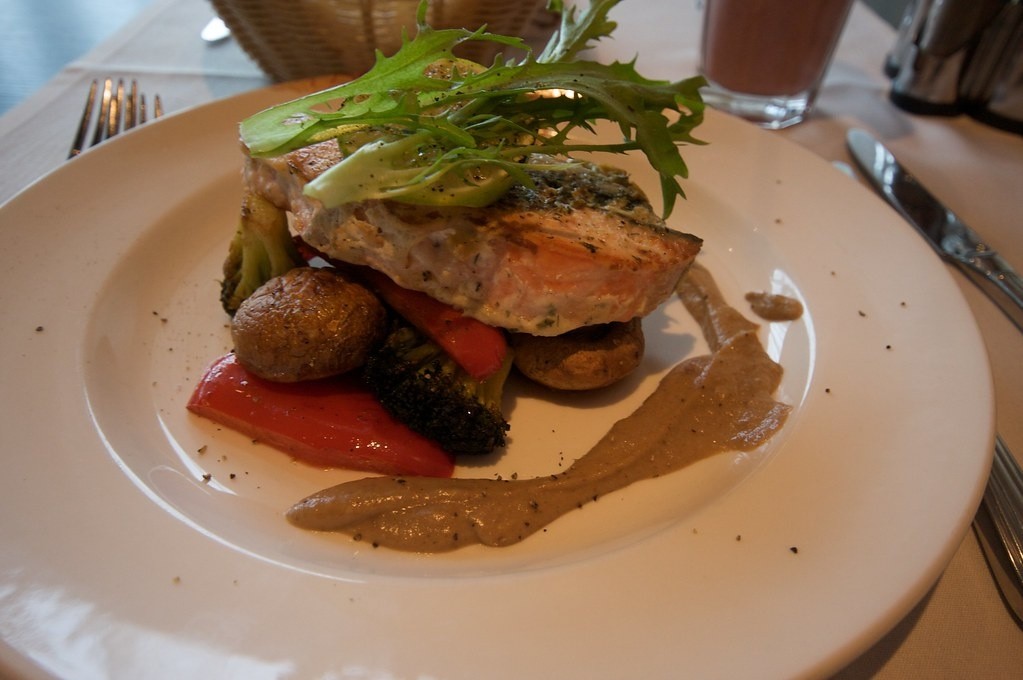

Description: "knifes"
844 116 1023 333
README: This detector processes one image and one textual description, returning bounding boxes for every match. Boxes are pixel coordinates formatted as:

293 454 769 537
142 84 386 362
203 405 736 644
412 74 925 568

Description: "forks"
66 74 164 160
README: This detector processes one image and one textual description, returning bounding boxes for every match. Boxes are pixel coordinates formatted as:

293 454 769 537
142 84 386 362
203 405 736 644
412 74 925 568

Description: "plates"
2 72 1000 680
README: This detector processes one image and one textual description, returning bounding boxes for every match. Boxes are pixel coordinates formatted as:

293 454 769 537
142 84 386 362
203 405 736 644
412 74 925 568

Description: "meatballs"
229 268 379 382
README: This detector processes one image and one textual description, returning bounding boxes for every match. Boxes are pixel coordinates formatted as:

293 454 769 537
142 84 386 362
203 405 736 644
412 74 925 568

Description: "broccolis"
367 320 510 458
219 190 300 322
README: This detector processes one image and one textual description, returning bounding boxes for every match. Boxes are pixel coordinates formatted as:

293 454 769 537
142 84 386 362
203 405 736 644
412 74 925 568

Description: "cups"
692 0 854 130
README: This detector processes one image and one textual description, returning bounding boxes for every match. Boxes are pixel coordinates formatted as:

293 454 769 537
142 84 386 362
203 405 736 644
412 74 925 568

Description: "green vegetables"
236 0 713 222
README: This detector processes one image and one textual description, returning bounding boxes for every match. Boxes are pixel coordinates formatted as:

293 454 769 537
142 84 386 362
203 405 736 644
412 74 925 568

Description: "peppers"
185 241 511 479
